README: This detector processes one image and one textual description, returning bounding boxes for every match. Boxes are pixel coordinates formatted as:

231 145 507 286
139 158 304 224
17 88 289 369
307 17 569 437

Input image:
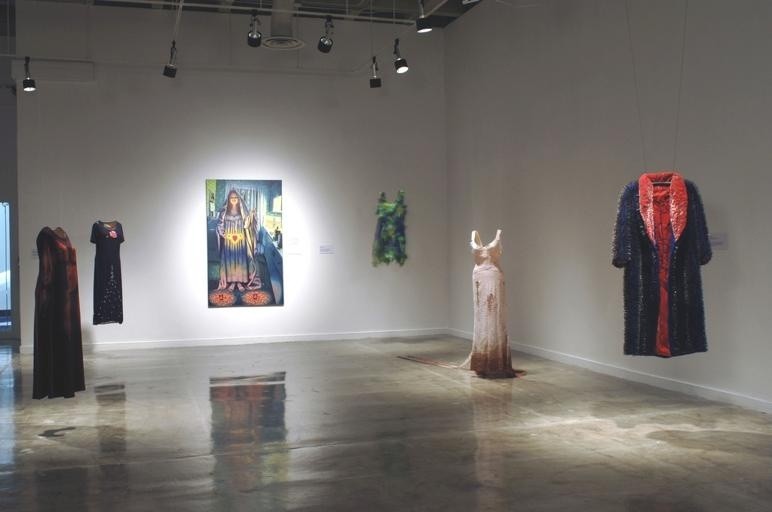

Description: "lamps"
245 0 435 88
154 41 185 79
14 55 42 94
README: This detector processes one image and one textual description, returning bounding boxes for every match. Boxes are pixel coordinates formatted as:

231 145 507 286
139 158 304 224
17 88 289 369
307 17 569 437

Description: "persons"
216 190 262 292
